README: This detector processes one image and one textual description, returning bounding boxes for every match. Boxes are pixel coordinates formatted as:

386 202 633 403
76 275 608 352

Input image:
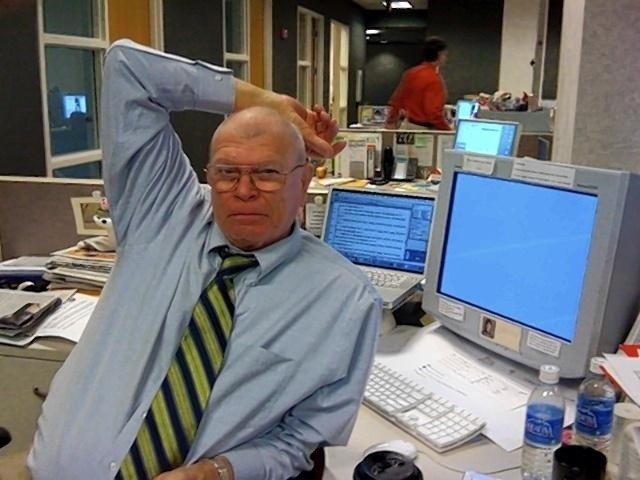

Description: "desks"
4 87 640 480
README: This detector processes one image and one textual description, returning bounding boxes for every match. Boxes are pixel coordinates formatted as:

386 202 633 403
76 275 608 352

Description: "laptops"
320 185 439 310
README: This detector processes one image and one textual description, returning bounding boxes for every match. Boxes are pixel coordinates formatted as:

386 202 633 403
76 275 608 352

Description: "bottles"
520 355 640 479
366 143 374 179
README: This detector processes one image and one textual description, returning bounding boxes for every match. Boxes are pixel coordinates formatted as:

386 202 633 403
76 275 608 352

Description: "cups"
316 166 328 178
528 95 538 113
464 94 476 103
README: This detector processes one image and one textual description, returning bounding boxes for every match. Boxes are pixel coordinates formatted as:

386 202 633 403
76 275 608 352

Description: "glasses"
203 165 306 192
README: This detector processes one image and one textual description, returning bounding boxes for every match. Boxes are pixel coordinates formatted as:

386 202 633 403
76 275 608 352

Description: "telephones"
383 146 418 182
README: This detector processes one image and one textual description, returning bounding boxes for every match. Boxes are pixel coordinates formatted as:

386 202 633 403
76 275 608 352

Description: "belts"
407 117 436 130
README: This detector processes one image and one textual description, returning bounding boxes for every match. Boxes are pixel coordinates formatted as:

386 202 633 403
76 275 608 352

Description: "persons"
385 36 453 131
480 319 493 338
26 38 383 480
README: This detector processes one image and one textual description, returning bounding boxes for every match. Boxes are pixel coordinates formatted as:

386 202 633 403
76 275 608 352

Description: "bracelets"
207 456 230 480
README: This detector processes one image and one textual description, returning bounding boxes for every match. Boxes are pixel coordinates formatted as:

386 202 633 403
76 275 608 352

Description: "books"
44 243 117 293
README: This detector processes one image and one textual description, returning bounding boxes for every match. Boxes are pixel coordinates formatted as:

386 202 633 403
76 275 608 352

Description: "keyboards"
362 359 487 453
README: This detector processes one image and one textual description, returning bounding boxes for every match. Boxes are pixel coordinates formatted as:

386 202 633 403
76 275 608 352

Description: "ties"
112 247 258 479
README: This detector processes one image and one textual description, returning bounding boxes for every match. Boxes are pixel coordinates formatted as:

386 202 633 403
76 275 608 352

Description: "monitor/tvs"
421 149 640 380
61 94 89 119
454 98 479 119
453 119 522 157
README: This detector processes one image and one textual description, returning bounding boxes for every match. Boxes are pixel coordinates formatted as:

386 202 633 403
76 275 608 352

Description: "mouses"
363 440 417 461
